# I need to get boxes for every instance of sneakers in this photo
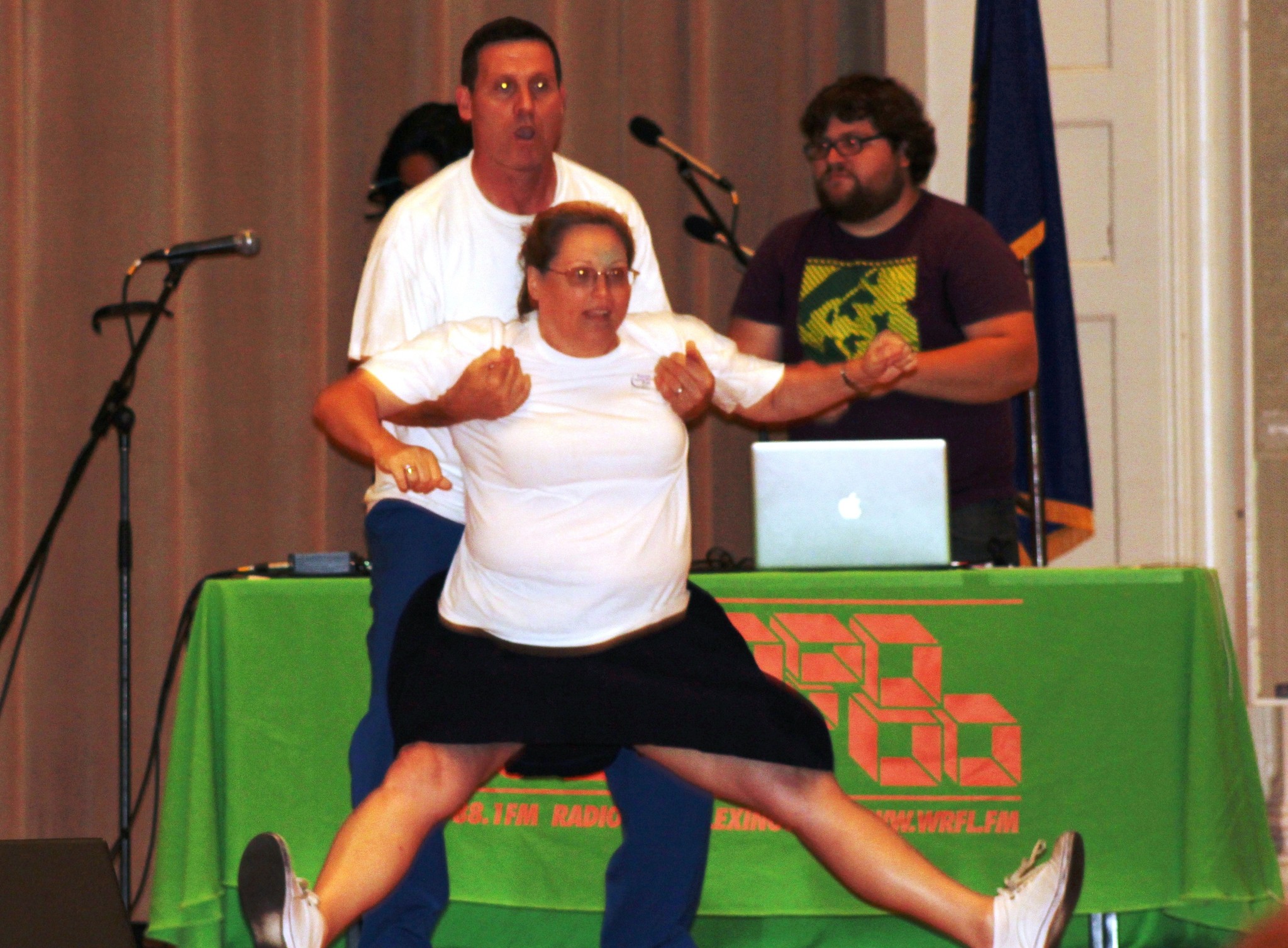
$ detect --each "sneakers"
[989,830,1083,947]
[240,831,329,948]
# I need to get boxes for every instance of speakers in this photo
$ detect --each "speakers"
[0,837,143,948]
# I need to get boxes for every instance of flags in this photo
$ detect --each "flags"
[965,0,1094,565]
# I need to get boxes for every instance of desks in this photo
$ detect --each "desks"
[143,569,1285,948]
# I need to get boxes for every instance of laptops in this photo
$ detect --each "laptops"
[749,437,965,569]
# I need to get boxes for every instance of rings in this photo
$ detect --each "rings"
[675,386,685,395]
[406,468,417,473]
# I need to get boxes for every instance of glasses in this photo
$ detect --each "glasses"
[469,77,554,99]
[801,132,882,161]
[541,266,639,290]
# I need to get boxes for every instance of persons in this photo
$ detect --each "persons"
[348,20,714,948]
[726,74,1038,564]
[239,201,1086,948]
[378,102,475,200]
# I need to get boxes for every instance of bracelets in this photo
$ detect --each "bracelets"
[843,368,863,396]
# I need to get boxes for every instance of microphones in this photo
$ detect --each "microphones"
[137,231,262,265]
[679,215,753,260]
[626,113,736,194]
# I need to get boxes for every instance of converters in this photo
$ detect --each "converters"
[291,549,364,576]
